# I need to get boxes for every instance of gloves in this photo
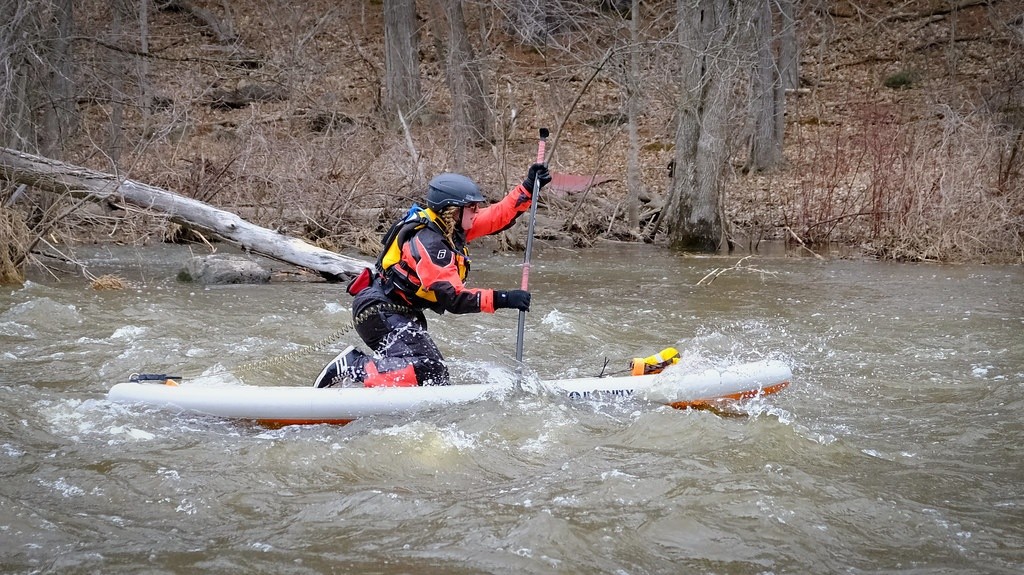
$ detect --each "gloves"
[494,289,531,312]
[523,162,553,192]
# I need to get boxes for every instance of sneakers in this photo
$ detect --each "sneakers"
[314,345,364,388]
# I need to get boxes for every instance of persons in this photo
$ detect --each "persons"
[302,156,553,387]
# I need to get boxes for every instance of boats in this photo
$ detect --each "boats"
[107,347,792,432]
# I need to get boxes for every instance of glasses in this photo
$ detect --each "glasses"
[466,201,480,212]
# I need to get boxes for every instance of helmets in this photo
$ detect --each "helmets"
[428,171,486,211]
